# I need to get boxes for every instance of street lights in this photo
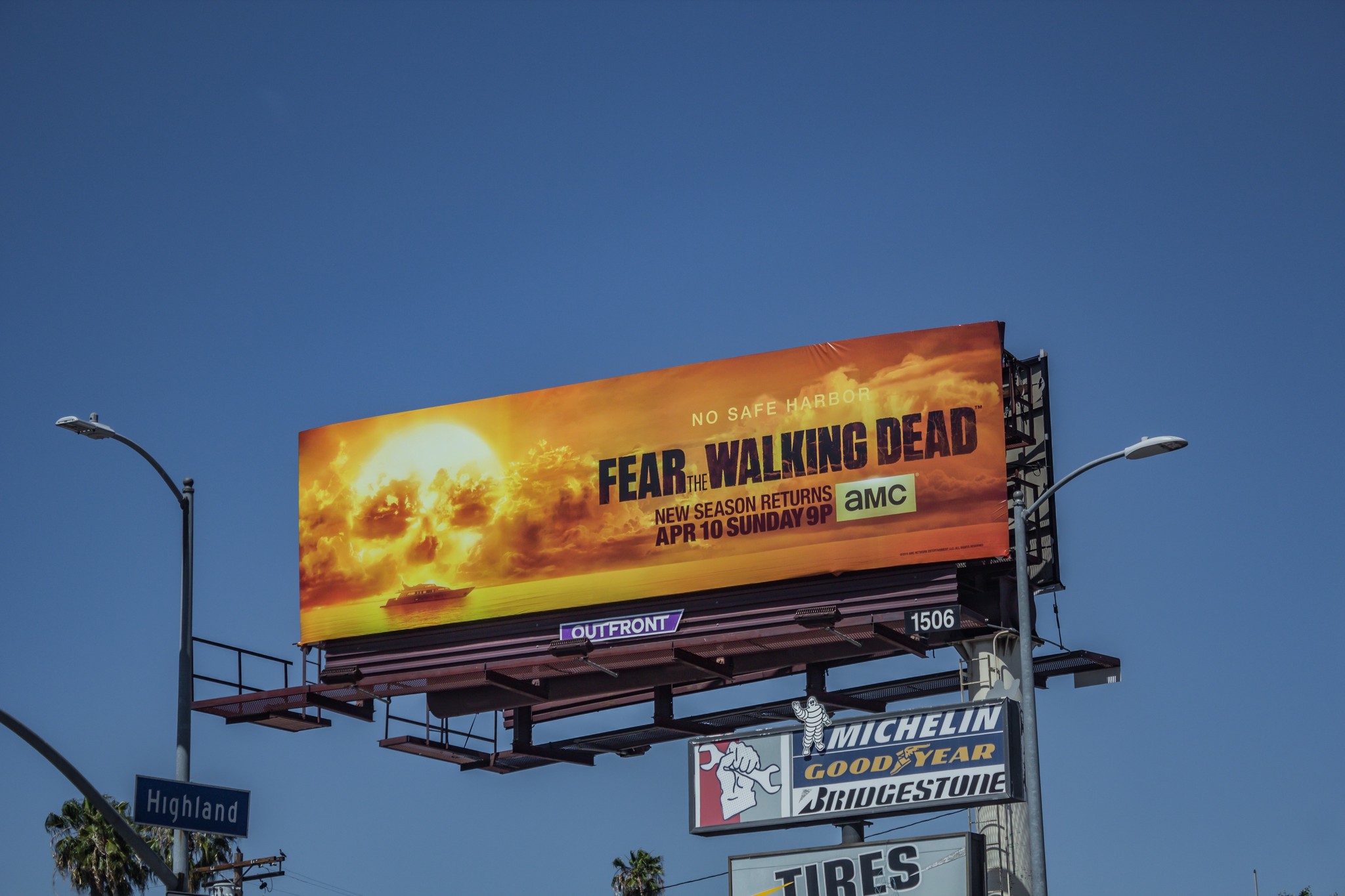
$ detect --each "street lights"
[55,413,199,896]
[1015,433,1193,896]
